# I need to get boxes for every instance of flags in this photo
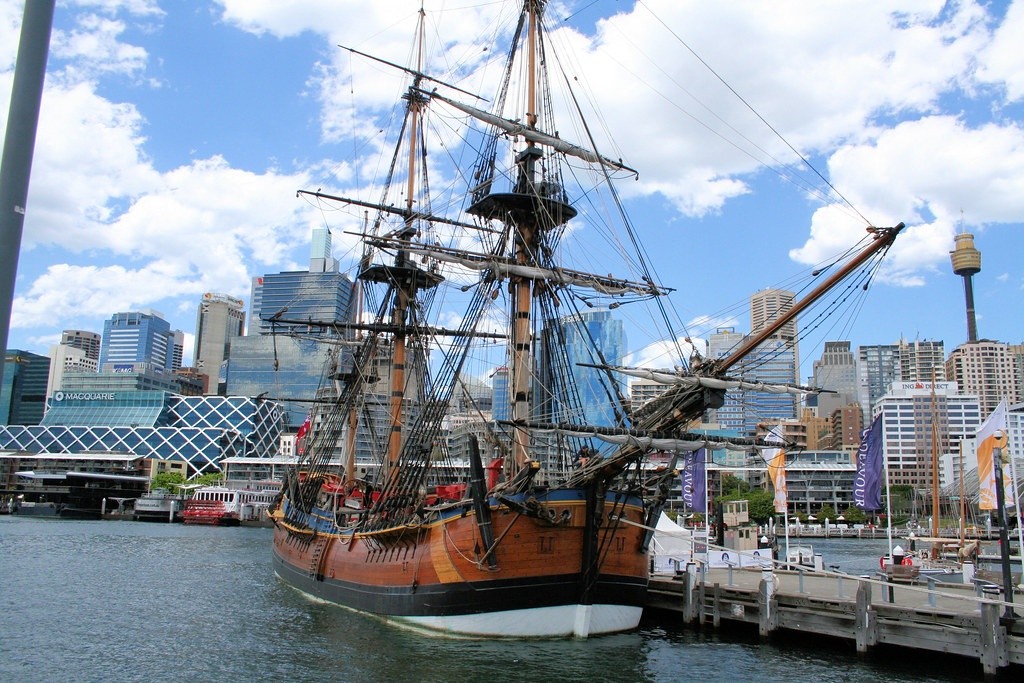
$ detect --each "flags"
[975,399,1015,510]
[682,448,705,512]
[762,424,787,506]
[853,412,883,511]
[295,407,311,446]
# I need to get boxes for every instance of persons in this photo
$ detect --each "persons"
[573,445,589,469]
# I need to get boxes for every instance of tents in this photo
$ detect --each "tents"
[648,511,691,578]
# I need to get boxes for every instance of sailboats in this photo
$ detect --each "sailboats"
[264,0,907,640]
[879,364,1021,595]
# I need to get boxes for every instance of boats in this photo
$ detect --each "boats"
[133,486,183,523]
[182,485,281,525]
[778,541,828,573]
[715,500,779,563]
